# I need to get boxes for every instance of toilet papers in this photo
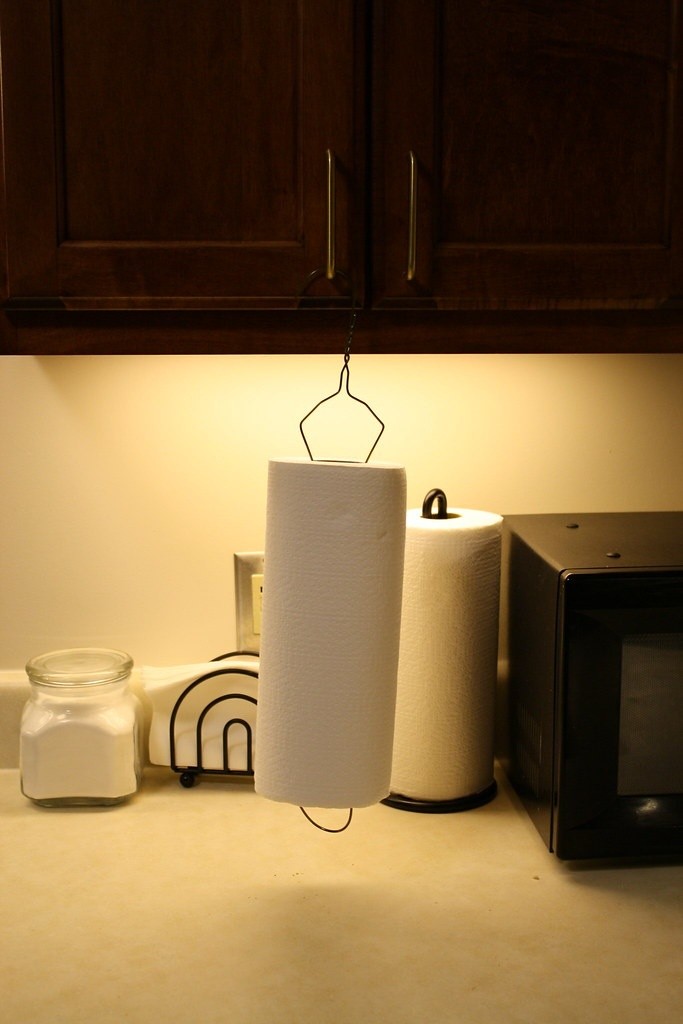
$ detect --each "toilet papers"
[389,507,504,800]
[255,458,406,809]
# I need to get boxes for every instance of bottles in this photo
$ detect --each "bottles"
[17,649,141,807]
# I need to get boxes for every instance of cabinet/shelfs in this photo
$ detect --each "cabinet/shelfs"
[0,0,682,354]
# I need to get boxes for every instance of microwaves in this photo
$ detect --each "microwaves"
[502,515,683,861]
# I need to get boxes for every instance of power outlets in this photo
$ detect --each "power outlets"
[233,552,265,651]
